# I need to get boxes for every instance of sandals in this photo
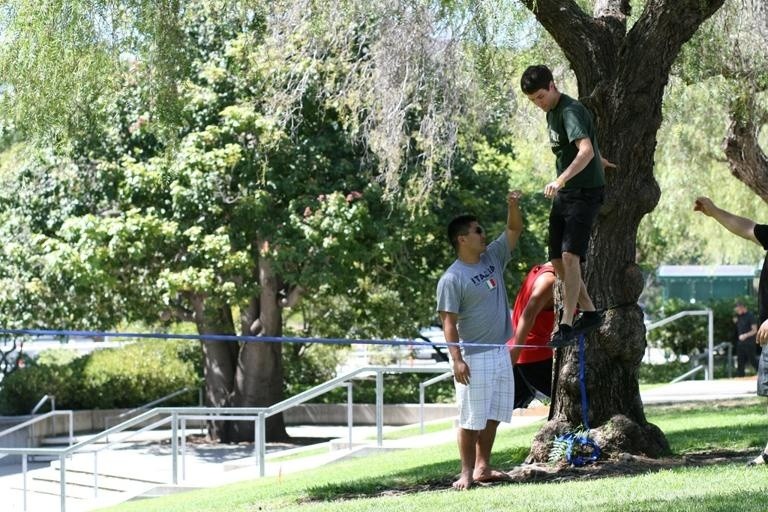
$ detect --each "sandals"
[745,451,768,467]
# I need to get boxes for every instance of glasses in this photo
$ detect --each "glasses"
[455,226,482,239]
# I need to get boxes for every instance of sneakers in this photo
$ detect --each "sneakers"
[545,324,578,347]
[567,310,602,336]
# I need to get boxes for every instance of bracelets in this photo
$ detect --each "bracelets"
[555,179,566,188]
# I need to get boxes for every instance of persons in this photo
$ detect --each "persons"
[507,259,560,415]
[518,62,620,351]
[692,193,767,467]
[431,188,526,491]
[728,298,761,379]
